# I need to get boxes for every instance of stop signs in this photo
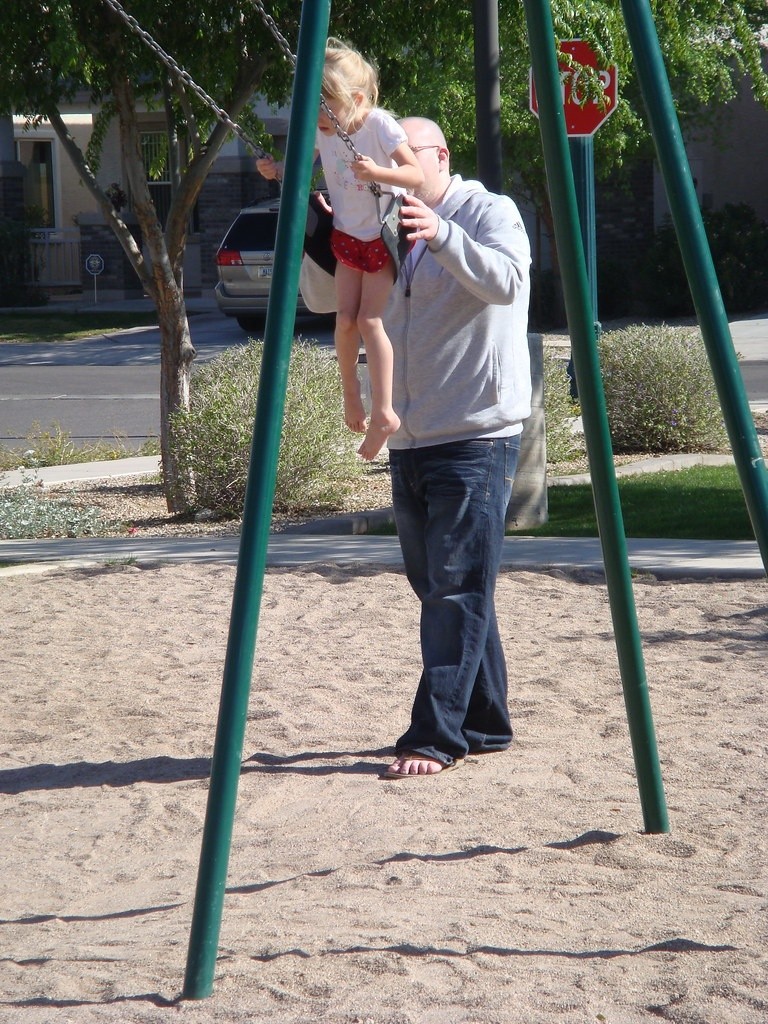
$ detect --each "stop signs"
[530,38,616,137]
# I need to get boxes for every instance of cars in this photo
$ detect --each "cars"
[214,191,337,334]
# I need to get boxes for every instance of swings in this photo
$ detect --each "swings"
[102,0,419,287]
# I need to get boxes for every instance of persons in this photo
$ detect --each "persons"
[253,45,424,461]
[381,113,536,776]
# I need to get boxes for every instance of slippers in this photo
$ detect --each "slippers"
[383,755,466,779]
